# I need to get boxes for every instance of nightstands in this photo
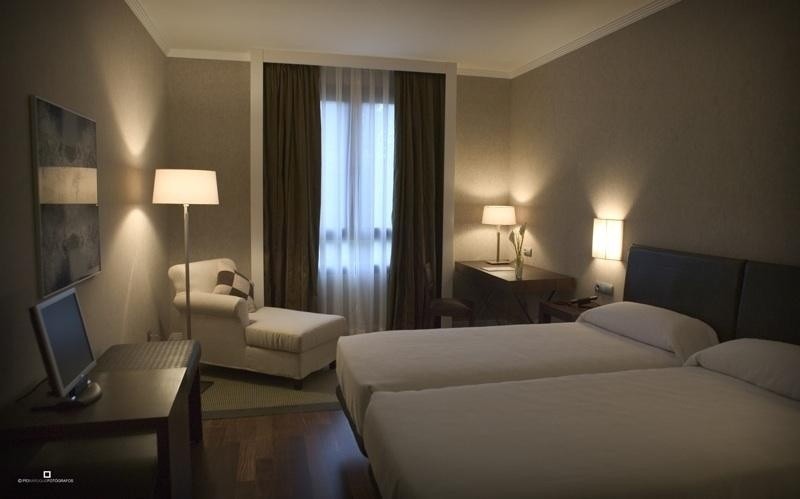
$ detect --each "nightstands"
[540,294,599,323]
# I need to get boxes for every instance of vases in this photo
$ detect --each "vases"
[513,254,524,280]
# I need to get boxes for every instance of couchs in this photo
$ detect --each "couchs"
[165,257,345,390]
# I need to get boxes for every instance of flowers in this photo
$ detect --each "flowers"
[509,221,533,270]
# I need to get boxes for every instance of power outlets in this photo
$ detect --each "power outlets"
[594,280,602,294]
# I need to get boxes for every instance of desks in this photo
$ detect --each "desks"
[452,259,577,326]
[3,339,204,499]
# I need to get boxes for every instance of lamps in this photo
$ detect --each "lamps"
[38,166,99,208]
[151,168,220,340]
[591,216,624,261]
[481,205,517,266]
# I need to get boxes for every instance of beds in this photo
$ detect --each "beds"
[362,258,800,497]
[327,247,800,456]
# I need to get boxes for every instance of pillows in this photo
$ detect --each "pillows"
[209,258,257,314]
[574,301,720,360]
[683,336,800,401]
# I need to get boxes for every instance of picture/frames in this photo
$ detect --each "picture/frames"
[30,95,103,299]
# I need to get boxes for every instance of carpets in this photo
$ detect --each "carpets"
[196,362,344,419]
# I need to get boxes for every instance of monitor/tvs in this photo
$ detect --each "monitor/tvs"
[29,286,102,410]
